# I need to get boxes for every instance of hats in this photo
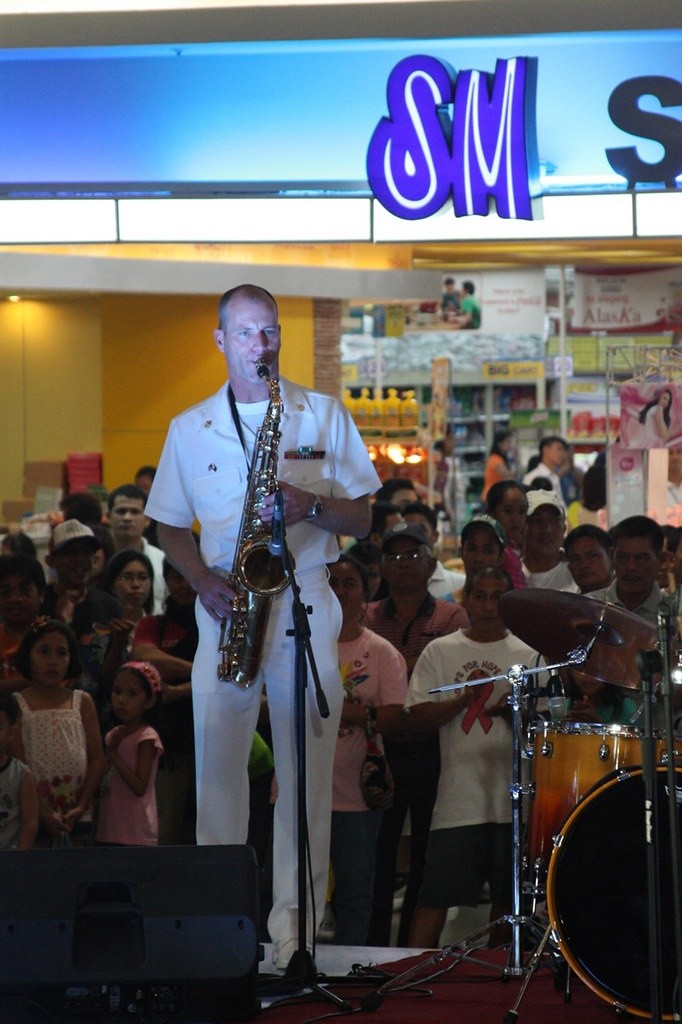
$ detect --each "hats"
[52,519,101,555]
[461,513,507,546]
[378,477,414,497]
[381,522,427,554]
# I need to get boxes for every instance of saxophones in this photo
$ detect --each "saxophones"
[215,361,295,690]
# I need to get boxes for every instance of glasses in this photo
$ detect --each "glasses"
[385,551,426,560]
[616,551,655,565]
[119,573,151,582]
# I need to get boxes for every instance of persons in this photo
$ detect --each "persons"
[143,284,383,972]
[0,429,682,950]
[627,389,673,449]
[441,277,480,330]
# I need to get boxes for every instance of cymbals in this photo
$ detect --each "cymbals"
[497,587,682,691]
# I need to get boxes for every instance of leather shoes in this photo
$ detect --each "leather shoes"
[271,937,297,969]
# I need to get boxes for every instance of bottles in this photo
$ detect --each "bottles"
[343,388,418,427]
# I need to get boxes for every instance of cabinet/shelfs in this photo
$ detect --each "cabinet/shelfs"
[354,413,514,563]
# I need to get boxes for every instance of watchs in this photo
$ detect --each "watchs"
[303,494,323,522]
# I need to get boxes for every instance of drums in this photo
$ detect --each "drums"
[520,721,682,896]
[545,762,682,1021]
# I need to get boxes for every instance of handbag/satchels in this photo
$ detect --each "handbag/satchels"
[360,703,394,811]
